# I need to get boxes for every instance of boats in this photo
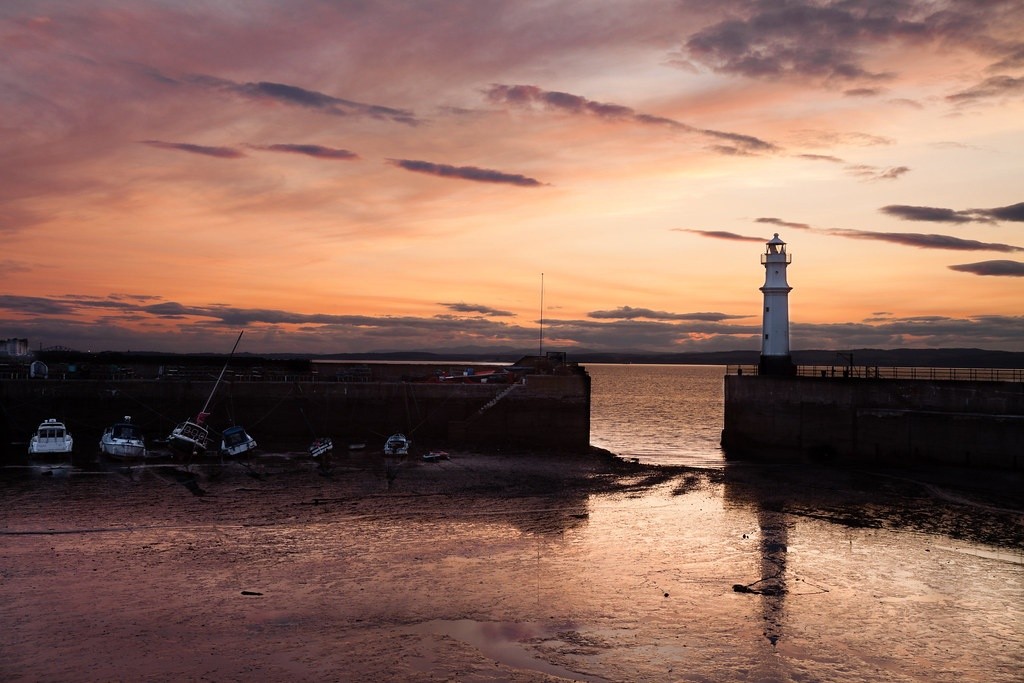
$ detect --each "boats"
[30,360,48,378]
[27,418,74,464]
[422,451,450,461]
[439,373,508,382]
[99,415,146,462]
[384,433,409,457]
[221,425,258,459]
[310,437,333,457]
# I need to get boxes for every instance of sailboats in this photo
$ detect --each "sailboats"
[164,330,244,462]
